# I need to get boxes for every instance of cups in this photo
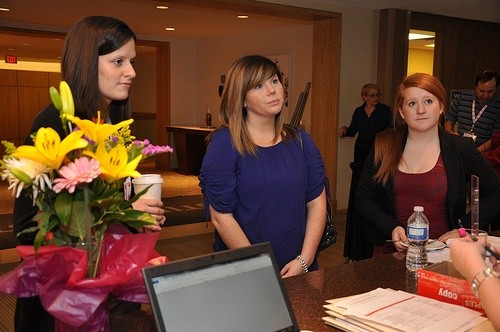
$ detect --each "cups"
[459,229,488,261]
[132,173,163,217]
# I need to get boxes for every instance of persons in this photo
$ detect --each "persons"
[197,54,331,279]
[356,70,500,260]
[12,15,166,332]
[338,83,398,163]
[450,235,500,332]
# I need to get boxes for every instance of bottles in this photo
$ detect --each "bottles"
[206,108,212,127]
[404,206,429,271]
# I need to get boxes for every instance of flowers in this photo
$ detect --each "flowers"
[0,81,172,253]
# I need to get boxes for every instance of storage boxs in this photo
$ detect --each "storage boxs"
[417,260,485,314]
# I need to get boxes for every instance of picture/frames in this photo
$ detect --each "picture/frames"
[266,51,292,108]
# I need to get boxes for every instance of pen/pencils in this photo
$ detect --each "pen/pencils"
[386,239,401,244]
[468,234,500,259]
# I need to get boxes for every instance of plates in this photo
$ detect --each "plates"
[399,238,447,251]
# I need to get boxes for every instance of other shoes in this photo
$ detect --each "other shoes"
[340,257,358,264]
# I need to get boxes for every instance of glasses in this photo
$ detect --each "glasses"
[366,93,381,97]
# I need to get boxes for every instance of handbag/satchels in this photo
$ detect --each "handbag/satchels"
[282,123,337,251]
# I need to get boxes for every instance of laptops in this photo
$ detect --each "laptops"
[140,241,301,332]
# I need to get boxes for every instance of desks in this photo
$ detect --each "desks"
[167,125,216,175]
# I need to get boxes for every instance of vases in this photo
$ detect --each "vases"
[66,242,102,278]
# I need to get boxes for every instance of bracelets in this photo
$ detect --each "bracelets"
[296,255,309,274]
[471,267,492,299]
